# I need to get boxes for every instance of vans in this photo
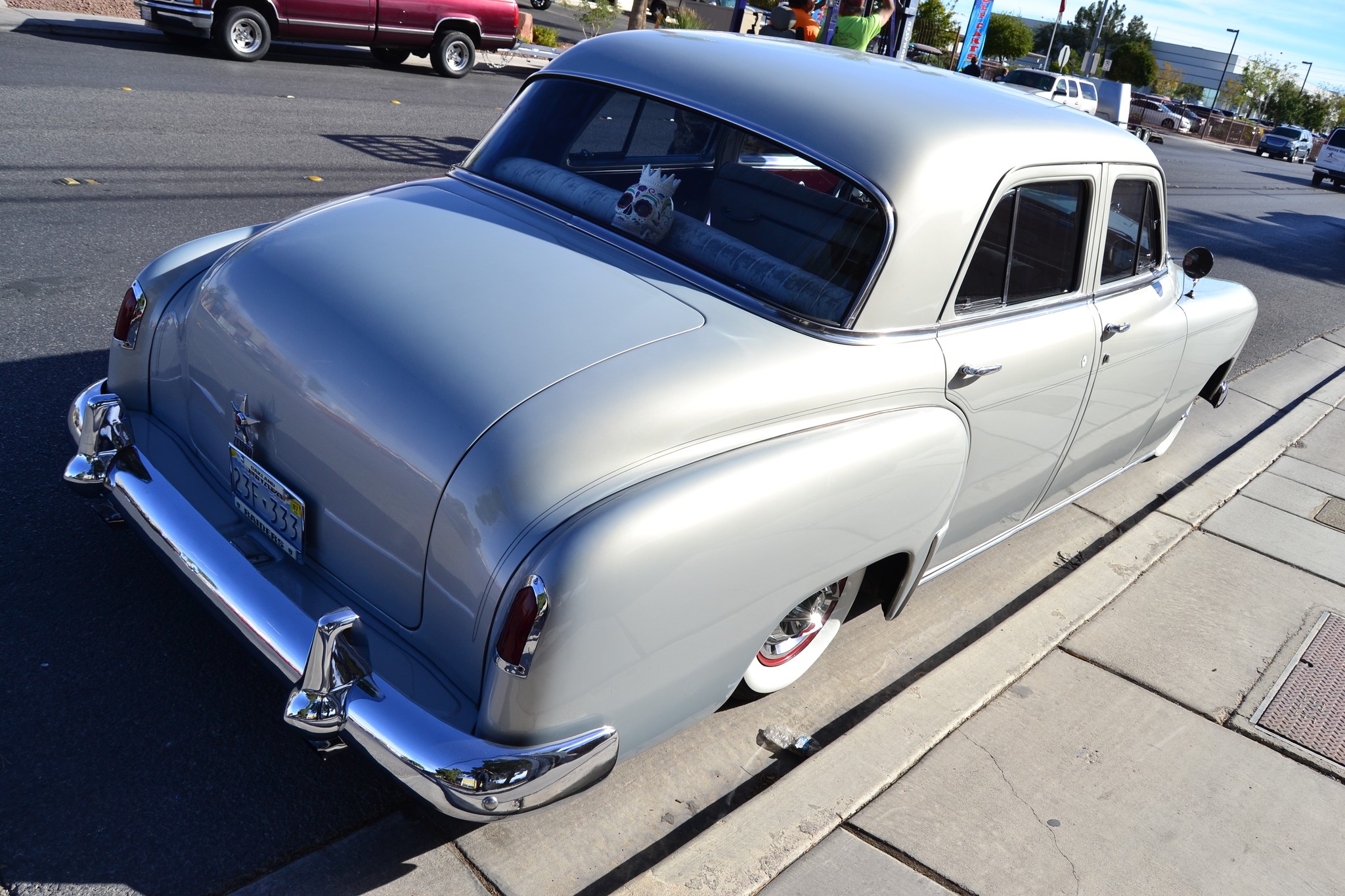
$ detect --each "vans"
[1311,127,1345,188]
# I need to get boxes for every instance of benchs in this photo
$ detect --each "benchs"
[491,155,856,328]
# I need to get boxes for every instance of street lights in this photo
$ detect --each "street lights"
[1201,29,1239,138]
[1297,61,1312,97]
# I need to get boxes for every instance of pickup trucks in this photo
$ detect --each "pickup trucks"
[647,0,771,33]
[134,0,534,79]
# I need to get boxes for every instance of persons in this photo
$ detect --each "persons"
[769,0,895,52]
[963,57,981,78]
[992,68,1009,82]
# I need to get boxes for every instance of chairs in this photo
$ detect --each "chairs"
[708,160,884,297]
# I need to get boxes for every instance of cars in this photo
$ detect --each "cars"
[64,30,1259,824]
[1129,92,1324,139]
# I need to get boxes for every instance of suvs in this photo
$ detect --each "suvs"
[1255,126,1313,164]
[996,68,1098,116]
